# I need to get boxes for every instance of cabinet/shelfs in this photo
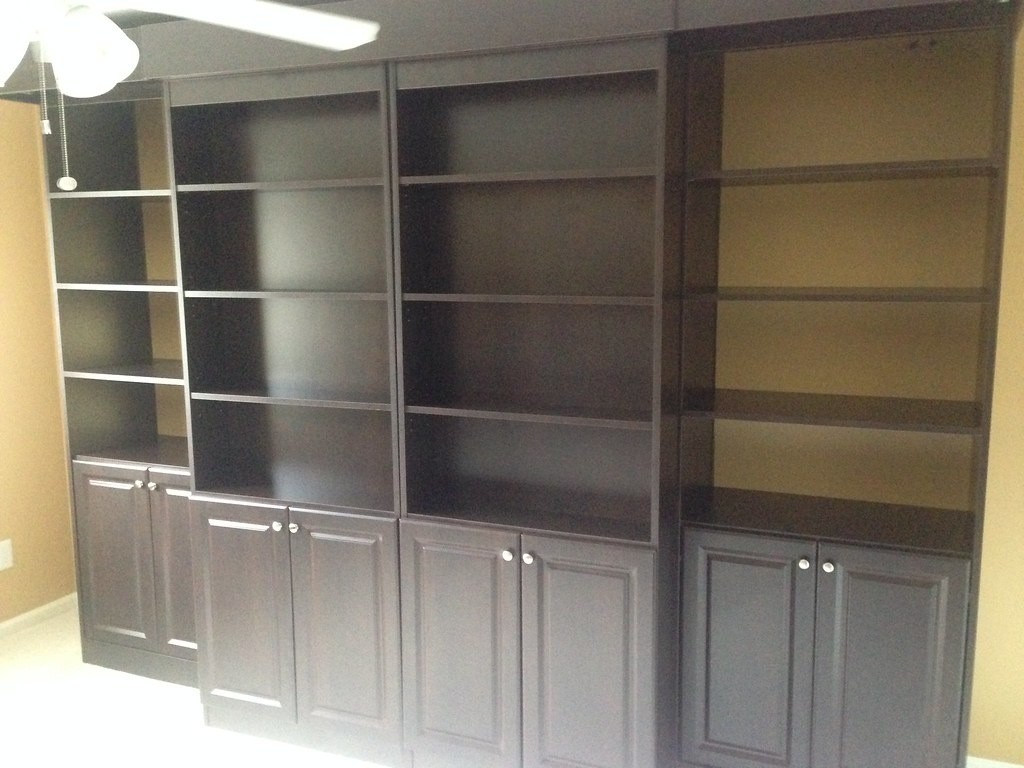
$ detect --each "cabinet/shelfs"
[163,57,397,758]
[38,75,199,688]
[676,4,1016,766]
[388,26,678,766]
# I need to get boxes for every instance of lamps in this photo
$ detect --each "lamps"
[1,2,382,100]
